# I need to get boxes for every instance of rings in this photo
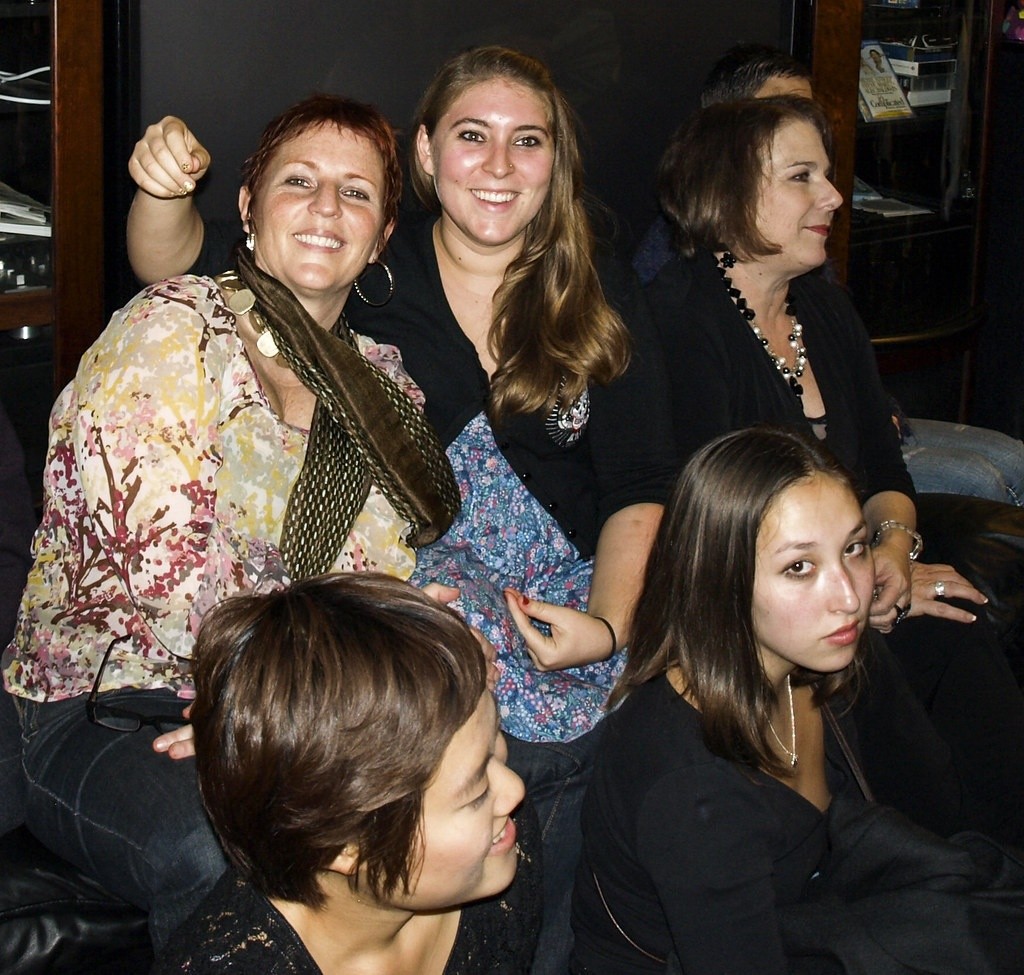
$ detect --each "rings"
[893,603,911,628]
[935,581,945,597]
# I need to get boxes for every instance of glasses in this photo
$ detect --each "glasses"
[85,633,193,735]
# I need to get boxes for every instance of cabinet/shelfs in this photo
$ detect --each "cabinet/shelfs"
[792,0,1000,424]
[0,0,103,419]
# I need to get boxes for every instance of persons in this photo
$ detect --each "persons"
[126,44,684,975]
[162,570,569,975]
[0,91,424,975]
[632,55,989,630]
[532,425,875,975]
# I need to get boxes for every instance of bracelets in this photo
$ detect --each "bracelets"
[873,585,880,600]
[595,616,616,663]
[869,519,924,563]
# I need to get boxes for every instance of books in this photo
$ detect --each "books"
[0,182,52,237]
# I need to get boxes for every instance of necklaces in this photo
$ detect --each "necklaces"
[711,256,809,396]
[761,674,799,769]
[219,269,290,369]
